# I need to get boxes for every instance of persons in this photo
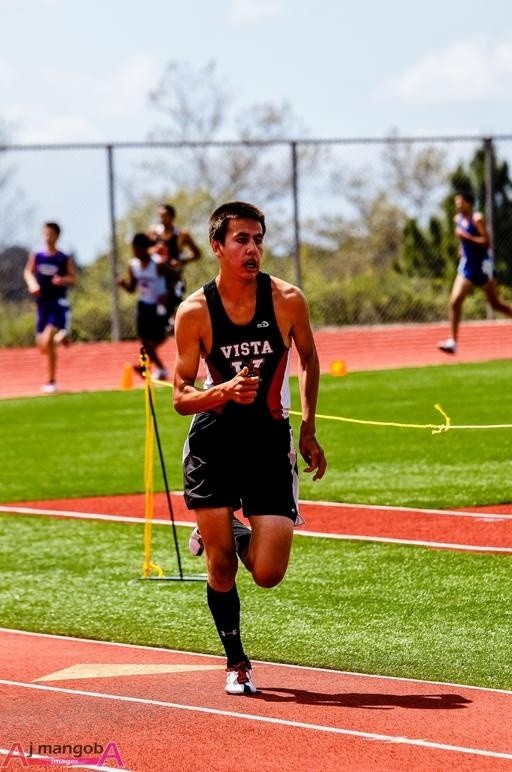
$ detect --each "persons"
[437,188,512,353]
[174,201,327,695]
[22,222,78,395]
[147,204,202,334]
[117,232,185,380]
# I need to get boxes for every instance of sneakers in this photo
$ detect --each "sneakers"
[226,661,256,694]
[189,527,204,556]
[439,338,456,354]
